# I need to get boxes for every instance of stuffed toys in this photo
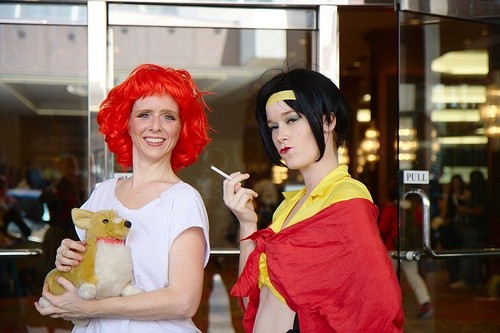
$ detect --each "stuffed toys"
[38,208,146,327]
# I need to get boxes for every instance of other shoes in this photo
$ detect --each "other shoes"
[417,301,435,323]
[450,278,470,290]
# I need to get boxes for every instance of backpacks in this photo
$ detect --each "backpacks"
[392,198,425,253]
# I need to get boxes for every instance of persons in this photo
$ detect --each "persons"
[0,157,87,237]
[223,68,402,333]
[444,171,488,239]
[378,181,435,318]
[35,64,218,333]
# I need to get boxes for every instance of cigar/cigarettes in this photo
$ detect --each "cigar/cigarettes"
[209,165,242,186]
